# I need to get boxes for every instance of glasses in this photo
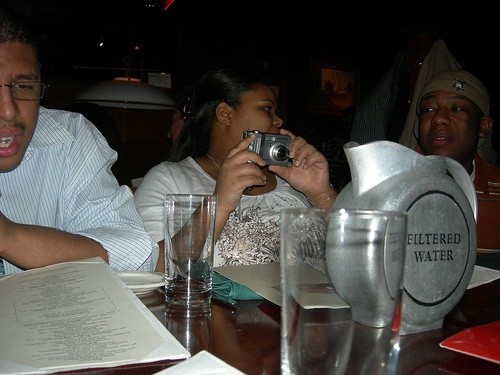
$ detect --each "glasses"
[1,80,49,99]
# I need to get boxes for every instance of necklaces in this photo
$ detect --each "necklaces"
[206,153,222,169]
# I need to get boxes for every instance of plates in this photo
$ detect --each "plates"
[118,271,165,295]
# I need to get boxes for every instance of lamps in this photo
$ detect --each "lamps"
[72,76,177,111]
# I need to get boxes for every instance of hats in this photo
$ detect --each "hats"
[416,69,491,118]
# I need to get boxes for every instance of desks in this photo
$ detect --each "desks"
[1,241,500,375]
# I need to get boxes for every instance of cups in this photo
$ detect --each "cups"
[164,192,216,319]
[279,208,407,375]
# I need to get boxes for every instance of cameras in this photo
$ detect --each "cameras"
[241,130,294,167]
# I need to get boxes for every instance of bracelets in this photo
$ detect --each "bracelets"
[306,184,333,206]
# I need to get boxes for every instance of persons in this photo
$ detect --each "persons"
[135,57,337,273]
[414,69,500,249]
[0,10,159,272]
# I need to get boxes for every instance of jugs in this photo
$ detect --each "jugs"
[325,140,477,335]
